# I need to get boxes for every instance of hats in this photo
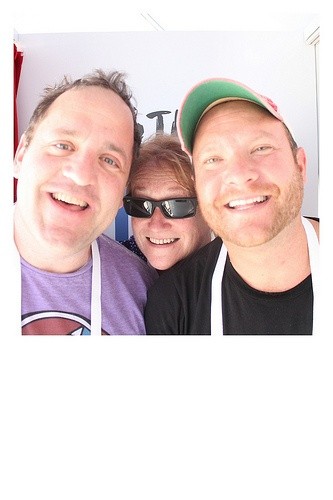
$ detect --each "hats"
[176,76,283,163]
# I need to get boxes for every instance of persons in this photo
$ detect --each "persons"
[142,78,320,337]
[14,68,158,335]
[120,129,215,272]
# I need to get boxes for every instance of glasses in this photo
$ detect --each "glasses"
[122,192,197,219]
